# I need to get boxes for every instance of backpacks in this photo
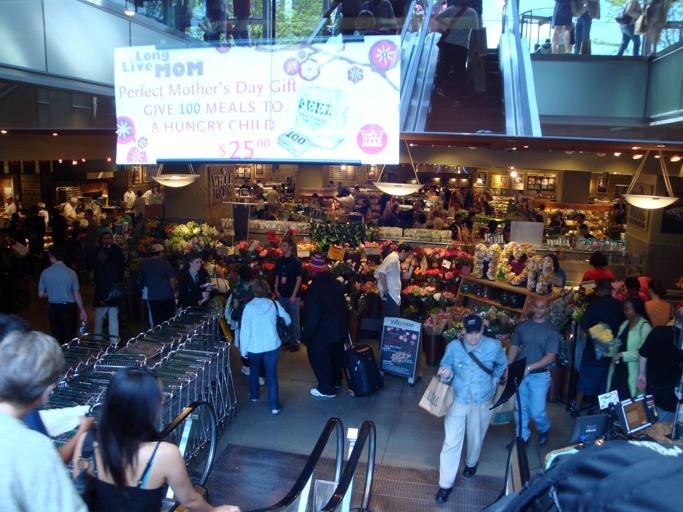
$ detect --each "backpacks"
[501,436,683,512]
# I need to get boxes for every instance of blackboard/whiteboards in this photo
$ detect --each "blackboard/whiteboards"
[380,317,422,384]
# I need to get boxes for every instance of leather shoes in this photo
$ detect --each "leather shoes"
[462,461,478,478]
[527,367,549,373]
[536,429,550,446]
[436,485,453,503]
[505,438,531,450]
[566,405,582,418]
[588,408,598,416]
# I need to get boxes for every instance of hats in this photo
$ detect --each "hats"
[151,243,165,253]
[307,253,326,273]
[464,314,481,334]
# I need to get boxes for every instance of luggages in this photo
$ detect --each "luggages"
[344,330,384,400]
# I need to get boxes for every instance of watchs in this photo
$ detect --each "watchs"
[528,365,532,372]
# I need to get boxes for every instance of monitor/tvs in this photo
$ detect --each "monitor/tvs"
[616,394,659,440]
[597,390,619,410]
[569,415,608,451]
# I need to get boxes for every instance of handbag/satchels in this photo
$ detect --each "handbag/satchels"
[418,372,452,418]
[272,298,300,354]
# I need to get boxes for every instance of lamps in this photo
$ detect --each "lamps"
[621,148,679,212]
[373,139,426,198]
[153,163,201,188]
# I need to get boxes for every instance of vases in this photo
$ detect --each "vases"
[423,300,432,310]
[406,297,416,306]
[370,296,380,316]
[348,314,360,343]
[426,335,444,368]
[124,296,138,321]
[548,368,562,403]
[139,297,147,322]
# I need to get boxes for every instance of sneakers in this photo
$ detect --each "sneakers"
[270,407,280,417]
[248,392,256,402]
[310,388,336,398]
[241,365,251,377]
[258,376,266,386]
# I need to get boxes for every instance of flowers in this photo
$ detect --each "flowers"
[337,276,367,314]
[164,219,234,280]
[588,321,622,347]
[124,257,144,296]
[526,255,554,294]
[499,241,536,285]
[418,286,435,297]
[546,297,573,369]
[234,238,315,275]
[448,305,517,335]
[422,307,451,336]
[361,281,379,294]
[401,286,419,296]
[472,243,502,280]
[346,237,397,273]
[103,271,139,302]
[434,291,458,306]
[403,248,472,285]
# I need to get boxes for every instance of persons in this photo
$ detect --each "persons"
[354,0,400,36]
[48,207,69,246]
[569,278,623,415]
[0,330,89,512]
[91,250,120,350]
[625,277,653,327]
[545,254,566,286]
[642,0,669,56]
[72,368,243,512]
[62,197,78,225]
[224,267,265,385]
[116,208,132,225]
[239,278,292,415]
[123,187,137,202]
[201,249,226,295]
[606,297,652,399]
[436,3,478,107]
[0,313,94,464]
[0,204,4,216]
[574,0,600,54]
[507,192,532,221]
[72,219,88,239]
[149,187,158,199]
[86,219,114,242]
[550,0,572,54]
[38,247,87,349]
[532,197,626,240]
[231,0,253,45]
[299,253,349,398]
[373,242,413,350]
[240,174,295,221]
[352,11,374,37]
[636,308,683,423]
[178,252,213,310]
[415,183,498,242]
[24,205,46,237]
[199,0,231,53]
[84,209,97,233]
[298,181,347,224]
[75,200,86,212]
[91,194,102,209]
[435,314,507,505]
[346,185,414,229]
[645,280,674,325]
[323,0,358,34]
[5,194,16,214]
[446,0,487,94]
[139,243,176,327]
[499,295,559,451]
[37,201,49,230]
[89,232,124,274]
[616,0,643,56]
[536,39,550,54]
[274,237,304,352]
[582,251,614,282]
[132,190,146,217]
[17,199,23,210]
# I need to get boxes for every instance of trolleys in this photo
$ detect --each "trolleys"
[36,310,240,466]
